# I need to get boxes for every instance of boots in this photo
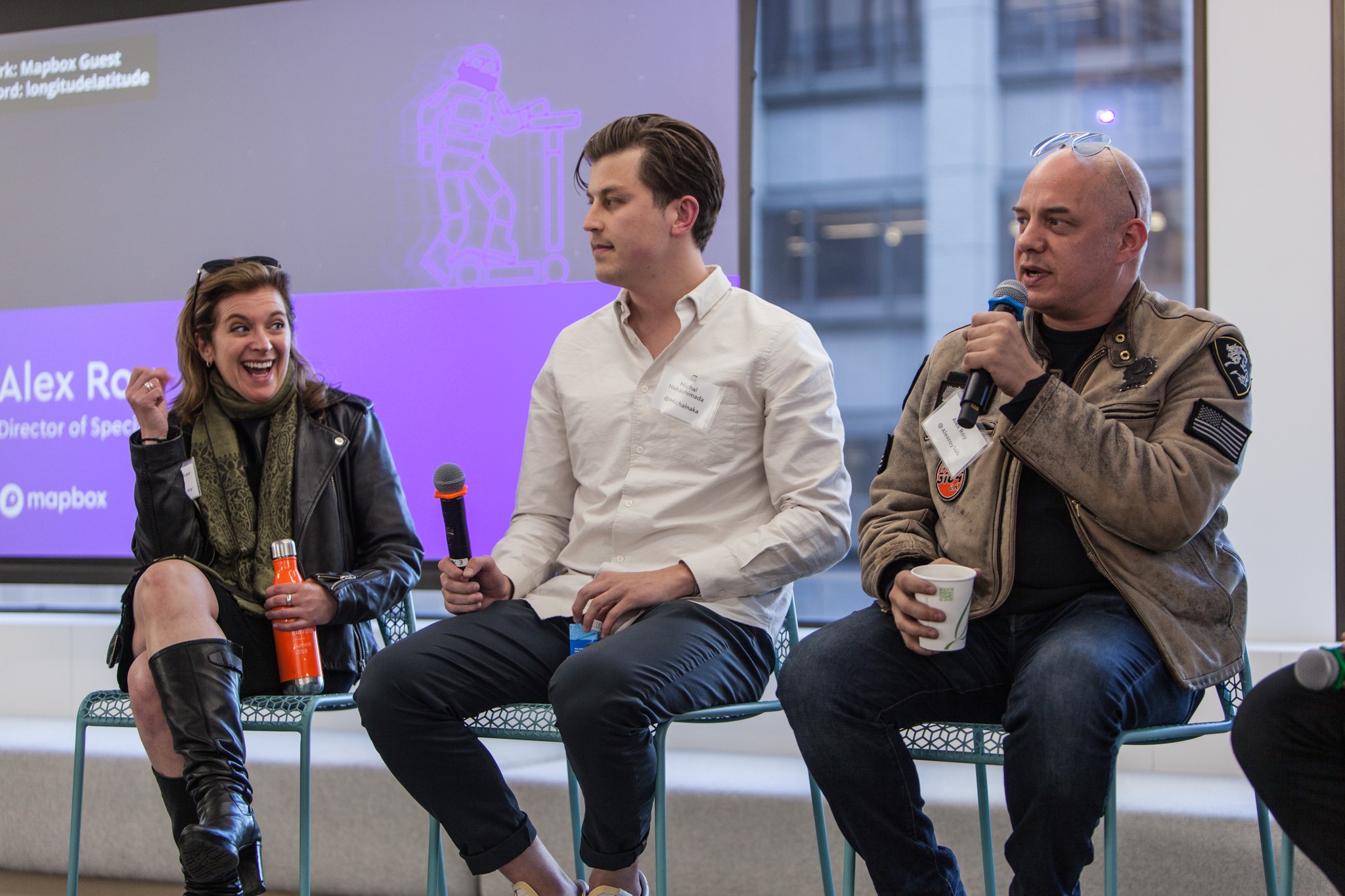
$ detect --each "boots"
[151,766,247,896]
[147,639,267,896]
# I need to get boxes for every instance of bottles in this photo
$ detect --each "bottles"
[270,538,325,696]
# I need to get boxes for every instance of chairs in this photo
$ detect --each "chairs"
[839,636,1281,896]
[67,591,447,896]
[424,589,838,896]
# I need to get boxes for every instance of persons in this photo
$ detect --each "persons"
[776,130,1253,896]
[1231,629,1345,896]
[352,114,851,896]
[107,255,424,896]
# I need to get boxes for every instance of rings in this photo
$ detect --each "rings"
[144,381,154,391]
[287,593,291,607]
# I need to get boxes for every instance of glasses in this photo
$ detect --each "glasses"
[191,256,283,348]
[1029,129,1138,221]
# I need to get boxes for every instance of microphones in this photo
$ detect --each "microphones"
[433,463,474,583]
[957,278,1029,429]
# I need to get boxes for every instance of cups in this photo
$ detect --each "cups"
[910,564,977,652]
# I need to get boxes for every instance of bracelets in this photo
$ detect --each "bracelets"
[140,438,167,443]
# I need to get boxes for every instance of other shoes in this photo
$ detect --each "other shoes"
[511,879,588,896]
[588,869,650,896]
